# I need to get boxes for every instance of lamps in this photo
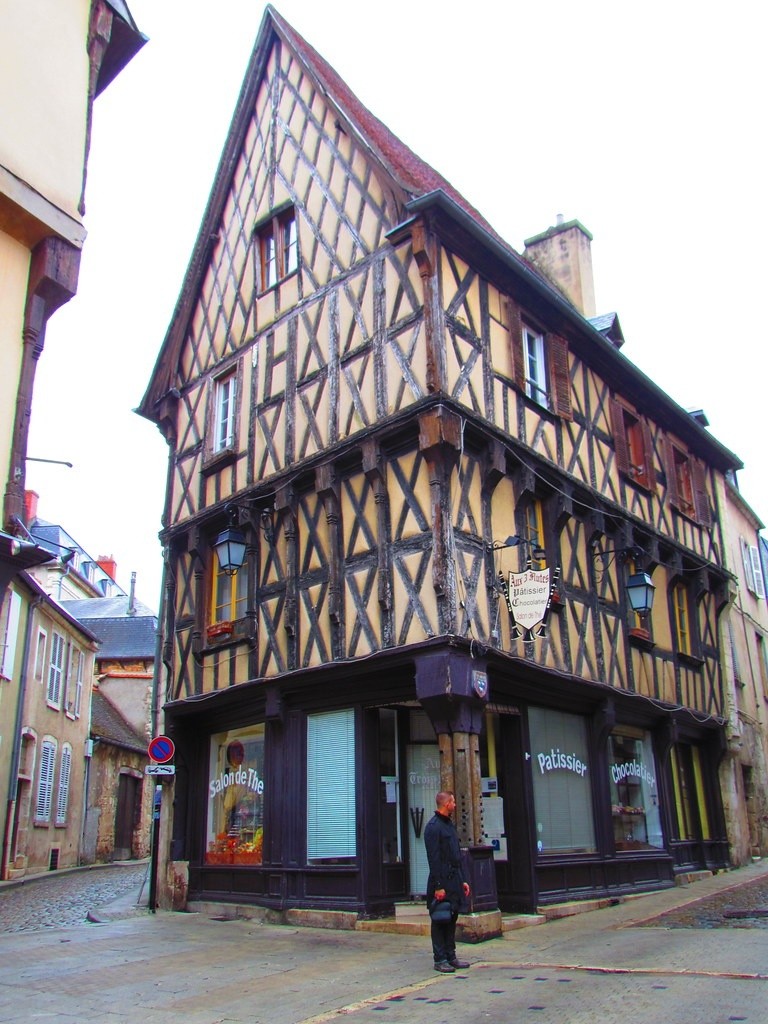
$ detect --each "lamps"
[214,503,273,577]
[592,546,657,620]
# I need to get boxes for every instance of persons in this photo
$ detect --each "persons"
[424,789,470,972]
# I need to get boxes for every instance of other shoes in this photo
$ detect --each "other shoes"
[448,959,470,968]
[434,961,454,972]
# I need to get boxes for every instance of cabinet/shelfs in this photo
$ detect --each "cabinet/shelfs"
[608,746,649,843]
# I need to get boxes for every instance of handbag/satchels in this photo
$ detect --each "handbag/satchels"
[430,897,453,924]
